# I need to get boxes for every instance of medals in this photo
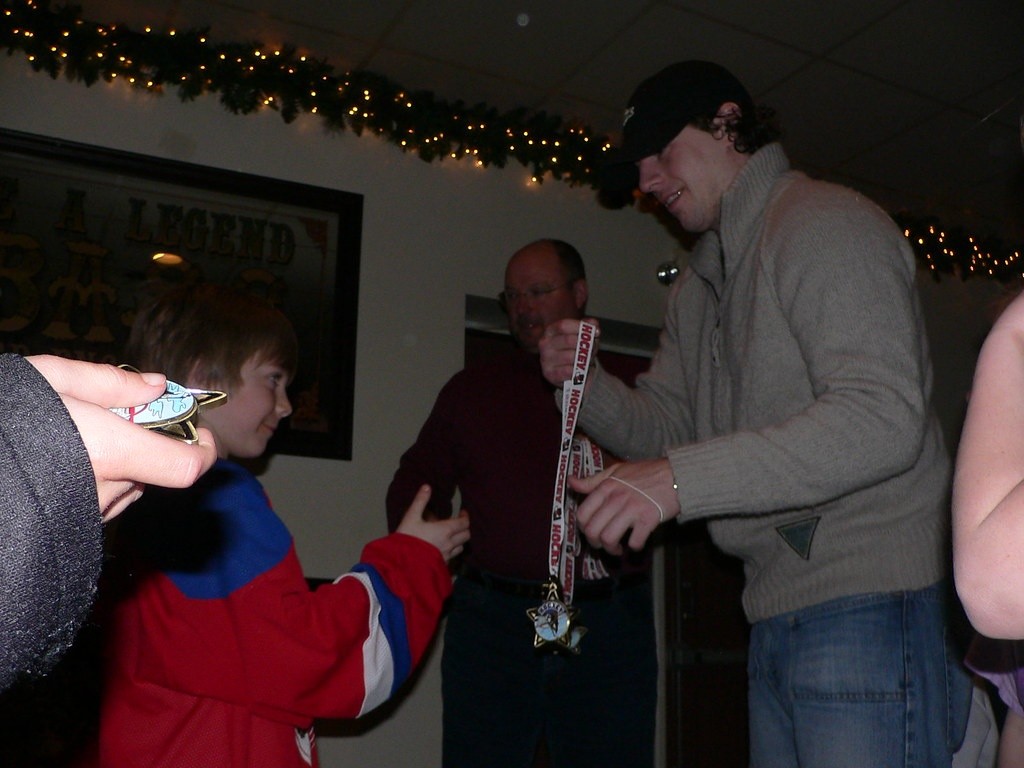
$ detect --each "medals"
[525,575,588,659]
[109,379,228,447]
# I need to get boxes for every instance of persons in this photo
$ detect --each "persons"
[536,57,956,768]
[0,350,220,708]
[385,239,658,768]
[950,275,1024,768]
[84,283,473,768]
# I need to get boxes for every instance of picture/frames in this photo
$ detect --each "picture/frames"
[0,126,364,461]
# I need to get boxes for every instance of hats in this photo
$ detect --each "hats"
[608,60,751,167]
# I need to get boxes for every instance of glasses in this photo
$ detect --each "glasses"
[496,278,579,308]
